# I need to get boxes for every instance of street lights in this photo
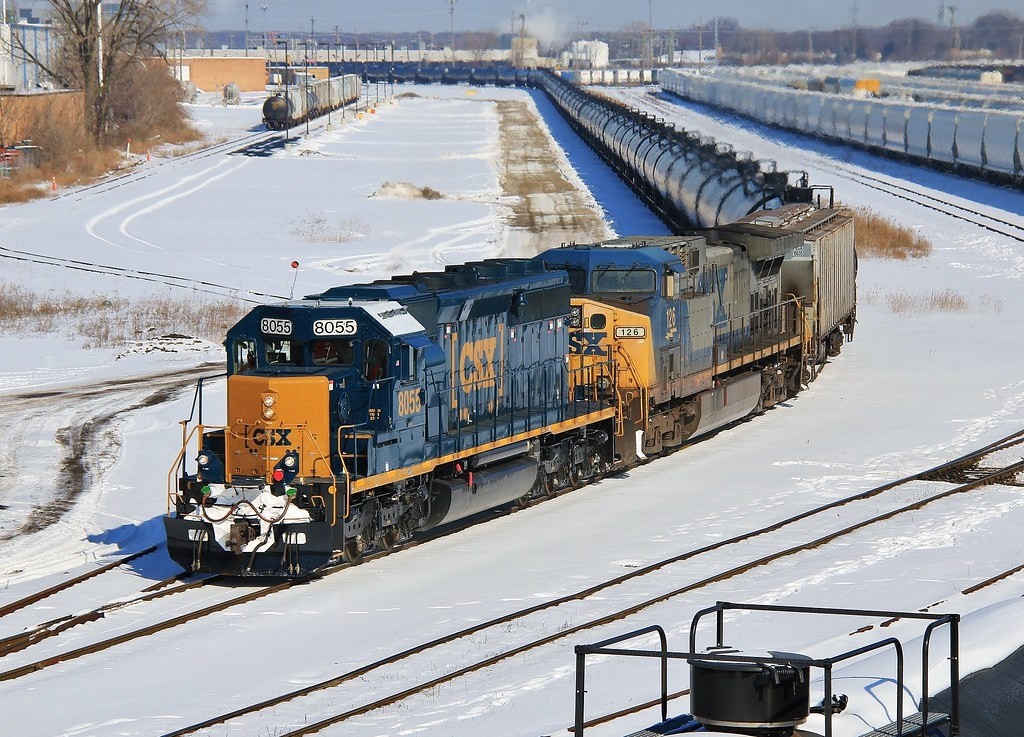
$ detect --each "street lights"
[334,42,346,125]
[260,4,268,46]
[360,42,369,112]
[319,41,332,132]
[276,41,291,152]
[349,43,359,118]
[370,43,378,102]
[297,42,311,140]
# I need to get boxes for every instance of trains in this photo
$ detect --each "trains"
[163,61,859,576]
[262,74,362,131]
